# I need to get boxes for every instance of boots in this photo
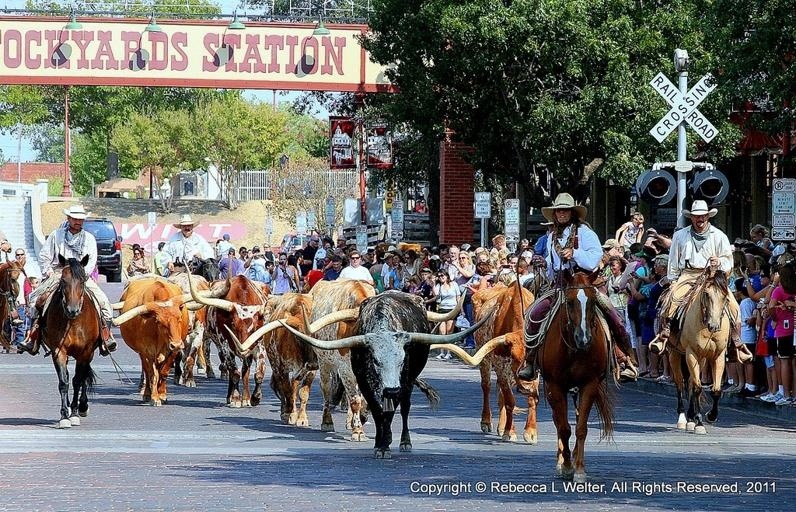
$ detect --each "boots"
[17,316,34,350]
[10,307,24,324]
[729,323,752,362]
[651,317,671,353]
[102,321,116,350]
[613,332,638,379]
[519,330,538,379]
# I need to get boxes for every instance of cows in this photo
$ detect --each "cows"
[110,252,540,459]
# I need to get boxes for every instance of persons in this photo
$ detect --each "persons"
[0,191,796,408]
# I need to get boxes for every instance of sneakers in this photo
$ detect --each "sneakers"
[639,370,796,407]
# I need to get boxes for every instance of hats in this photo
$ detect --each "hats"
[62,205,91,220]
[635,249,656,261]
[380,252,394,260]
[602,239,620,249]
[173,214,199,229]
[540,193,588,225]
[682,200,718,218]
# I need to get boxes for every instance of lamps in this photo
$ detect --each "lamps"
[228,11,245,29]
[313,14,329,36]
[145,12,160,32]
[65,8,82,29]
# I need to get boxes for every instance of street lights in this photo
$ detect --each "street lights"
[673,48,690,233]
[59,85,72,196]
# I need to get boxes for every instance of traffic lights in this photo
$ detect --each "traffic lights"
[692,169,730,206]
[639,170,677,206]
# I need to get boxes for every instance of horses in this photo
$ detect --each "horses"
[537,267,617,484]
[0,257,30,354]
[42,254,100,429]
[654,266,734,435]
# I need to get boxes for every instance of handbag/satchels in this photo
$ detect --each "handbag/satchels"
[455,315,470,328]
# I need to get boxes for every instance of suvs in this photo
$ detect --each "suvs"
[75,217,124,284]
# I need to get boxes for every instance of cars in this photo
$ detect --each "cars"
[282,179,318,199]
[281,234,314,252]
